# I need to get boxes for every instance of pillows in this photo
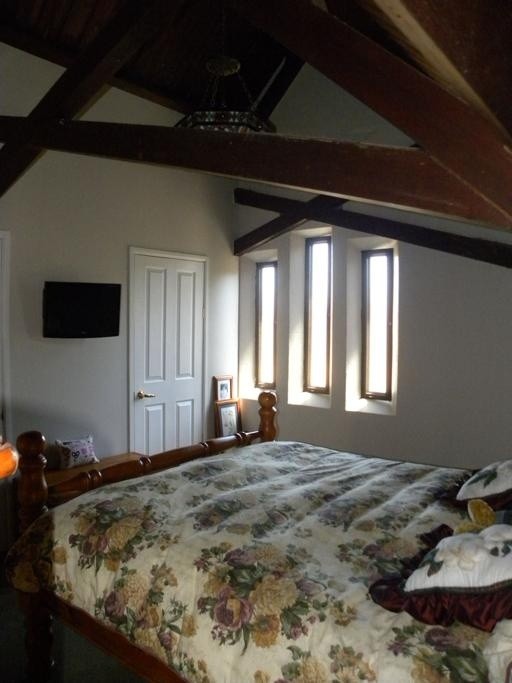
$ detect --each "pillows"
[373,518,512,628]
[56,435,97,469]
[453,458,512,510]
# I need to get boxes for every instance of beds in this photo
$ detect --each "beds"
[5,392,512,682]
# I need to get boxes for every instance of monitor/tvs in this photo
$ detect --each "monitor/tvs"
[42,281,121,338]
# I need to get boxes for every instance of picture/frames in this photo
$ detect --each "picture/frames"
[213,376,232,401]
[214,399,242,437]
